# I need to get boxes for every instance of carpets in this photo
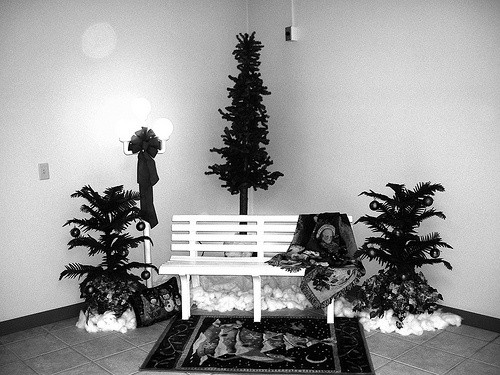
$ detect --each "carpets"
[138,309,376,375]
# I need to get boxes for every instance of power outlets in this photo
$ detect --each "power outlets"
[284,25,298,43]
[38,161,51,182]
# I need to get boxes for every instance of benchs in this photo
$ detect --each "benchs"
[157,212,355,325]
